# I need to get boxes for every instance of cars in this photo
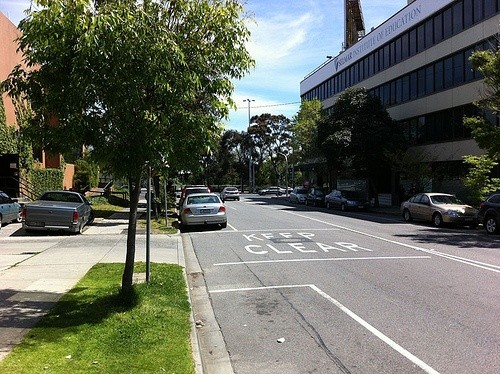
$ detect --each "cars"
[0,191,22,231]
[258,186,286,196]
[177,185,211,208]
[324,189,371,212]
[290,186,309,204]
[400,193,480,229]
[179,193,227,232]
[305,186,331,207]
[220,186,239,201]
[283,187,294,194]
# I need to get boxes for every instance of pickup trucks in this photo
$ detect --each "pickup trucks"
[21,191,95,235]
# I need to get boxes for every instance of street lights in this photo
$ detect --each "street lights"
[243,99,256,194]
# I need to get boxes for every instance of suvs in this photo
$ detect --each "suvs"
[479,193,500,235]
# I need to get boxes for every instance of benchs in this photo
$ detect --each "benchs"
[189,198,214,204]
